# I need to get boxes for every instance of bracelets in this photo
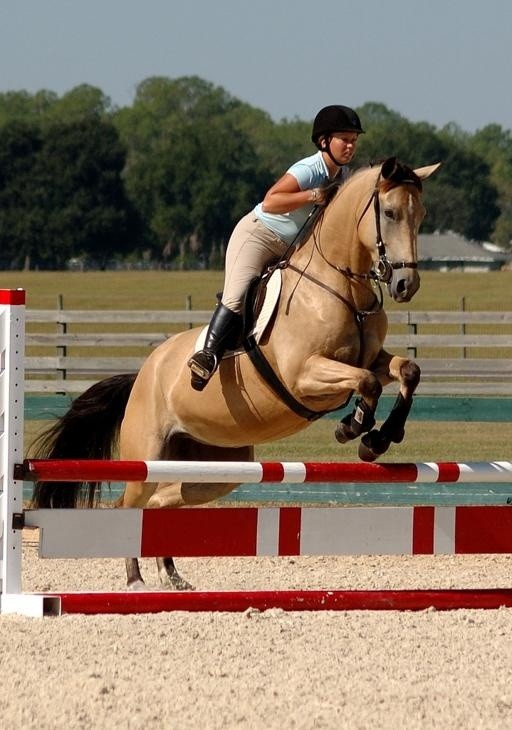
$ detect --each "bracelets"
[307,187,320,204]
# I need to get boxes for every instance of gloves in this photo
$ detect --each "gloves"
[308,176,340,208]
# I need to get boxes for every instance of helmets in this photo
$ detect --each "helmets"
[311,104,366,144]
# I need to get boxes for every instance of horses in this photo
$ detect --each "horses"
[23,156,442,591]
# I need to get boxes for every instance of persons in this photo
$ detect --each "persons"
[188,103,356,396]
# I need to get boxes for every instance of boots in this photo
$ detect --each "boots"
[190,299,242,391]
